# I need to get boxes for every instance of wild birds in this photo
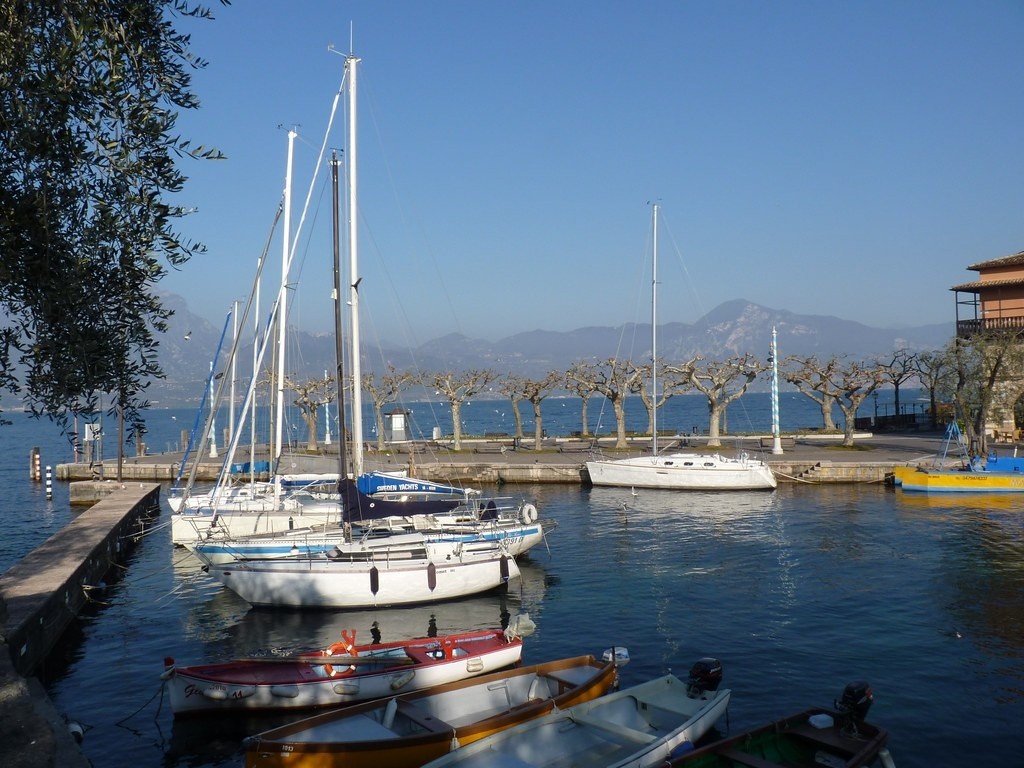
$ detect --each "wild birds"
[183,331,193,342]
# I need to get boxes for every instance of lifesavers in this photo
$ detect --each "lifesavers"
[323,641,359,677]
[522,504,538,525]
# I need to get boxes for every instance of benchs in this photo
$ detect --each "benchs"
[560,439,602,454]
[243,444,292,455]
[527,439,557,449]
[799,428,825,432]
[396,444,427,454]
[324,444,351,454]
[571,431,593,436]
[412,441,440,451]
[760,438,795,452]
[611,431,634,434]
[572,714,661,745]
[703,429,723,434]
[647,439,682,454]
[523,432,535,437]
[485,432,508,438]
[714,747,785,768]
[656,430,677,435]
[396,700,455,732]
[405,648,435,663]
[474,441,515,455]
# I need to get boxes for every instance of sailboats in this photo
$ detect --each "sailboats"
[584,196,778,490]
[167,18,559,607]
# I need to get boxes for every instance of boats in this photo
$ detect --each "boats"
[159,612,536,719]
[885,412,1023,493]
[242,645,630,768]
[653,680,896,768]
[418,656,733,768]
